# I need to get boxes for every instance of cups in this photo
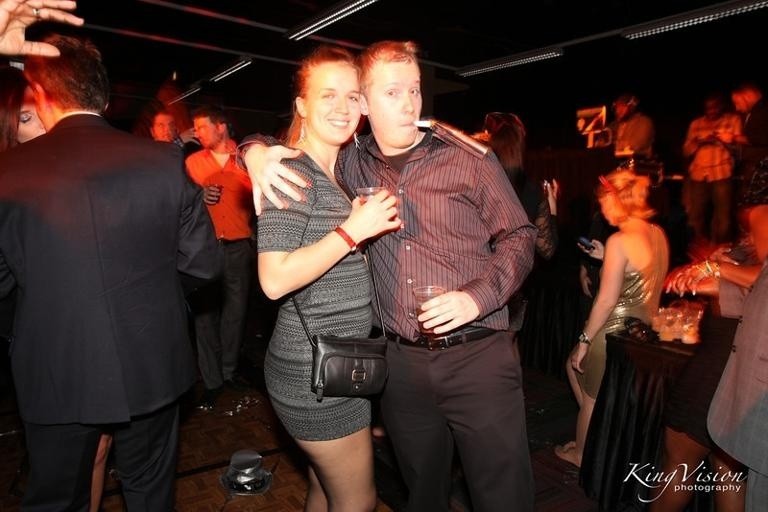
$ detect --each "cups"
[409,284,446,338]
[354,183,396,235]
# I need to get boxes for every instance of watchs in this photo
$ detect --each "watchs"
[579,332,593,347]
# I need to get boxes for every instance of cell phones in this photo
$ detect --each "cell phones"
[579,236,595,250]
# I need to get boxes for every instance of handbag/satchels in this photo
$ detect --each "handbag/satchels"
[312,335,389,397]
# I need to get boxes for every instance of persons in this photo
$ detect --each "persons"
[465,109,560,397]
[652,84,767,512]
[0,31,220,512]
[234,38,543,512]
[149,111,201,149]
[0,0,88,59]
[0,71,113,512]
[550,166,671,468]
[253,43,402,512]
[613,94,656,159]
[184,107,253,408]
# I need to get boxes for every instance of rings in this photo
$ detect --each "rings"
[34,8,43,21]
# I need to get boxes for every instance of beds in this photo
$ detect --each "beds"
[578,302,713,512]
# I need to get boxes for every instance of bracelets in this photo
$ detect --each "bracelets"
[238,143,252,169]
[335,226,359,252]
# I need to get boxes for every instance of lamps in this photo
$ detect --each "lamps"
[453,44,564,78]
[619,1,767,38]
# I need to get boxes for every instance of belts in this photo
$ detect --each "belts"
[371,325,498,348]
